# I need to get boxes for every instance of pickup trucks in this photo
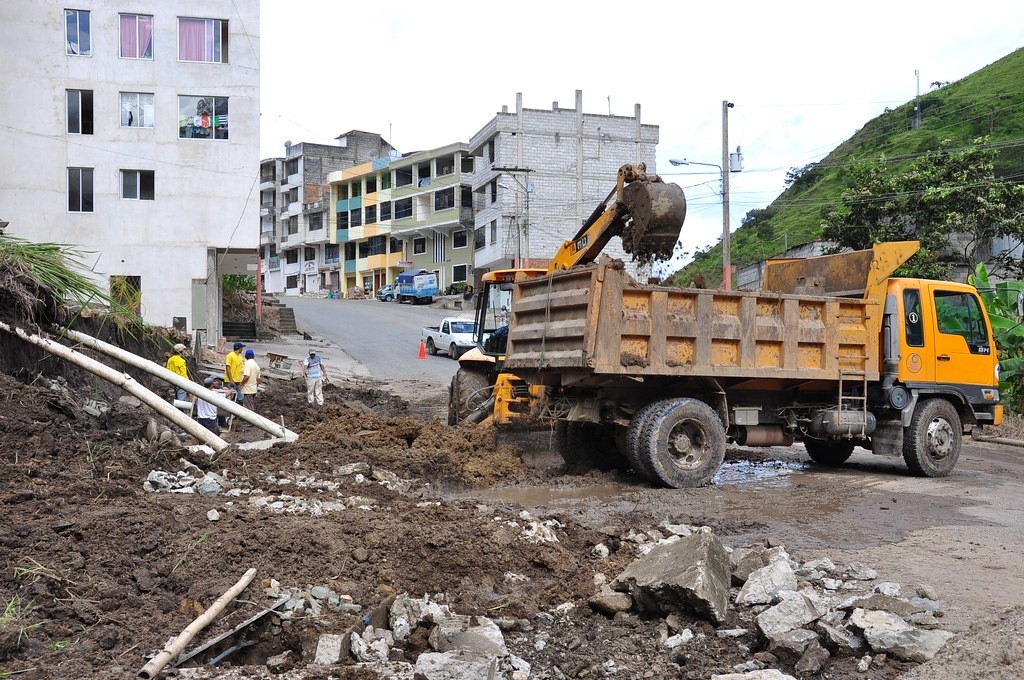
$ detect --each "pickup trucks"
[421,317,490,360]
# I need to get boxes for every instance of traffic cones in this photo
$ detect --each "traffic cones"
[416,340,428,358]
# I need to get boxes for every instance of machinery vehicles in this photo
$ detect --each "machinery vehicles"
[448,162,687,424]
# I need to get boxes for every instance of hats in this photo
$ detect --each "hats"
[309,348,316,355]
[210,375,218,380]
[174,344,186,352]
[203,378,214,386]
[233,342,246,349]
[245,349,254,357]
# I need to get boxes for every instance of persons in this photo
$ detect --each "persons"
[303,348,329,405]
[237,349,260,427]
[166,344,190,412]
[224,343,246,406]
[209,375,229,427]
[197,378,235,436]
[497,305,508,326]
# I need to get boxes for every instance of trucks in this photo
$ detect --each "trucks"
[394,269,437,305]
[503,240,1004,488]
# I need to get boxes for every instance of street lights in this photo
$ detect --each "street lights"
[498,182,529,269]
[669,158,731,293]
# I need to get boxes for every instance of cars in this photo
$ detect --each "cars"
[377,285,395,301]
